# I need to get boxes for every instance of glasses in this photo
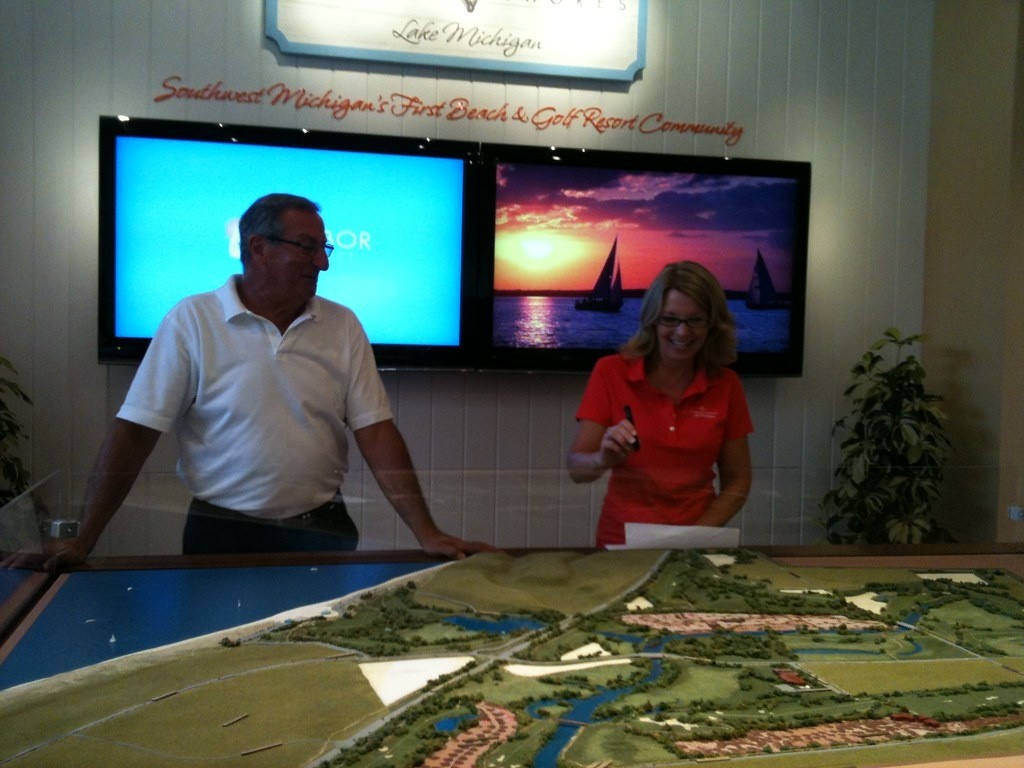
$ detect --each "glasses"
[268,237,334,259]
[656,315,712,328]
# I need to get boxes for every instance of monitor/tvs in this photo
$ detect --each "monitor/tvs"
[97,115,478,373]
[477,141,811,379]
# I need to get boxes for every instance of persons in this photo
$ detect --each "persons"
[0,193,505,571]
[567,260,752,549]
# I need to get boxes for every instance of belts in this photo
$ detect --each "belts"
[190,498,335,526]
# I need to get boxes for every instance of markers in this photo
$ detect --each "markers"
[624,404,640,452]
